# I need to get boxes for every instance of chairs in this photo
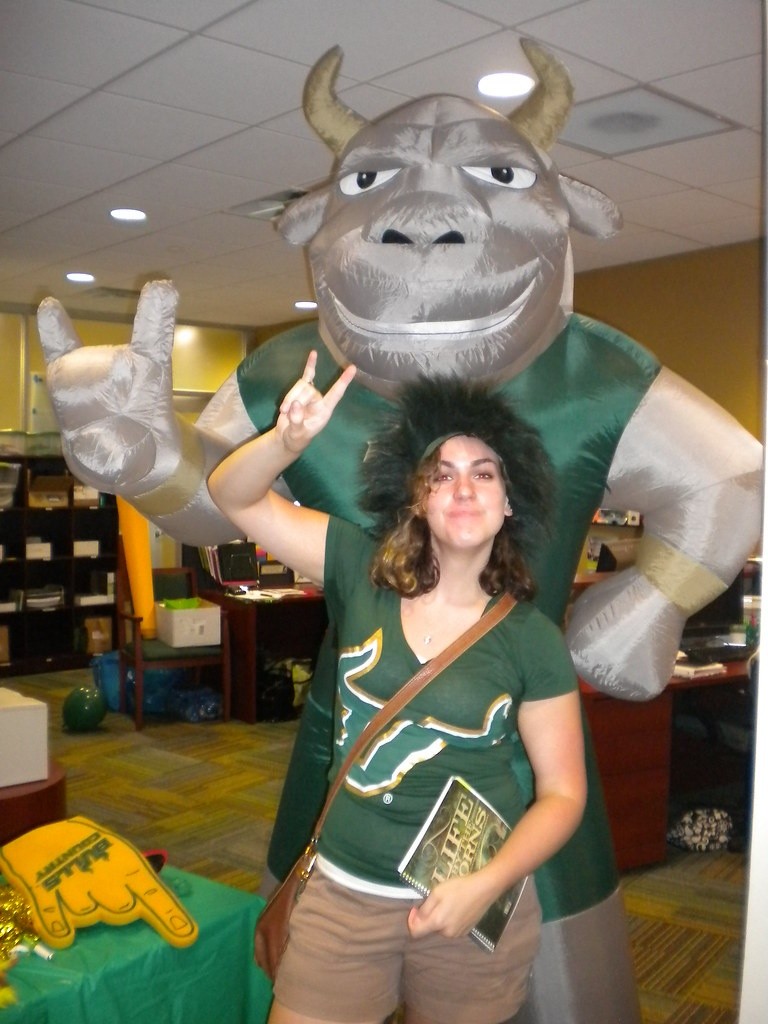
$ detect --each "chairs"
[116,568,231,731]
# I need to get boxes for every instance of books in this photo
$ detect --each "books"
[397,776,529,953]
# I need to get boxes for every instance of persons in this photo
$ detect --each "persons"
[206,349,587,1019]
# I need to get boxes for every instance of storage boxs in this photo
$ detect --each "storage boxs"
[26,537,42,544]
[83,615,113,654]
[0,488,14,512]
[0,461,22,490]
[26,543,53,561]
[0,624,10,664]
[28,432,62,455]
[73,539,100,558]
[28,476,71,508]
[0,429,28,455]
[155,596,221,649]
[626,510,641,526]
[742,595,762,627]
[0,686,48,788]
[74,479,99,509]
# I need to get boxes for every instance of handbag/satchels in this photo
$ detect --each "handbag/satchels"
[253,843,318,982]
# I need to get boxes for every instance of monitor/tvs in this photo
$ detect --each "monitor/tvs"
[682,568,744,638]
[595,538,641,572]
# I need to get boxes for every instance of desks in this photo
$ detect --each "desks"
[202,581,324,723]
[0,863,274,1024]
[573,571,623,604]
[575,659,752,879]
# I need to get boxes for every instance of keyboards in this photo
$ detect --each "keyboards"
[688,645,752,663]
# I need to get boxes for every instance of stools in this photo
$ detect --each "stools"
[0,759,69,847]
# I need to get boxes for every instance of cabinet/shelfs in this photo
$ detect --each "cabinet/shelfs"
[0,455,118,681]
